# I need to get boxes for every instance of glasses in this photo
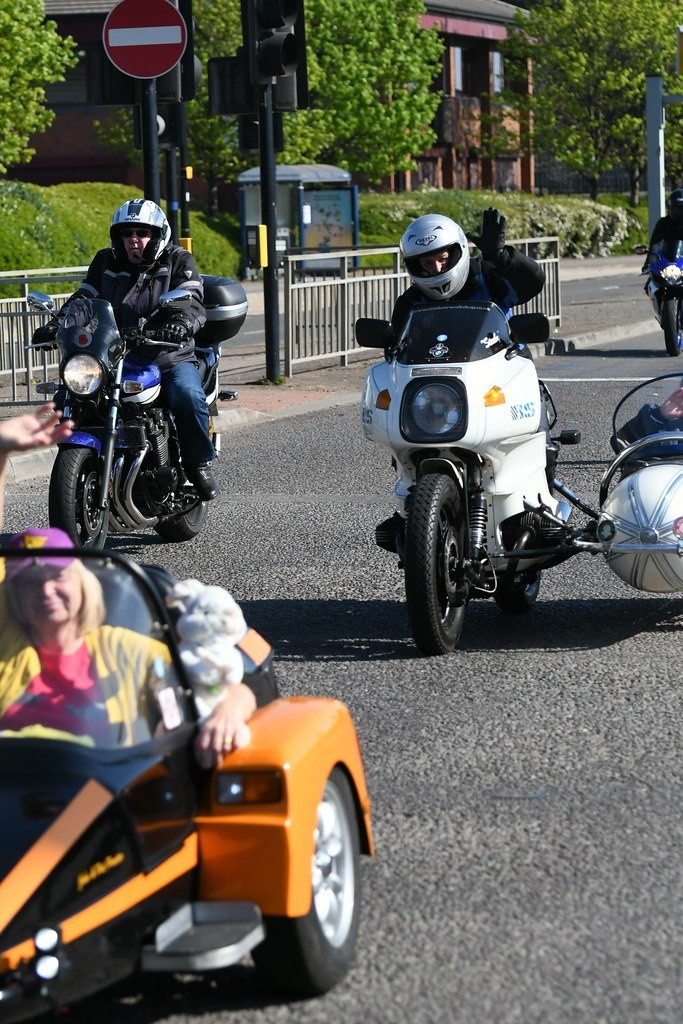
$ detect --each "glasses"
[119,229,150,237]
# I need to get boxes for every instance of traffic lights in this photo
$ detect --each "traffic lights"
[240,0,303,77]
[177,0,203,101]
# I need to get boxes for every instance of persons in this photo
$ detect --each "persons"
[0,402,258,769]
[386,207,553,496]
[31,198,218,500]
[642,189,683,295]
[610,386,683,455]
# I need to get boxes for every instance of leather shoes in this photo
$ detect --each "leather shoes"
[184,467,220,501]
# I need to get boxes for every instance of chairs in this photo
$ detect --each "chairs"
[94,569,155,635]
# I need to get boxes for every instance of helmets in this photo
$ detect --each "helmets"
[665,189,683,218]
[110,199,171,263]
[400,214,470,301]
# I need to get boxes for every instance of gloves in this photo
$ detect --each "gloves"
[154,321,187,344]
[642,263,649,275]
[465,207,506,261]
[32,322,59,352]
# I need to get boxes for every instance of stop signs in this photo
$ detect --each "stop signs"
[102,0,187,78]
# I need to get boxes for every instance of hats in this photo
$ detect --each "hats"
[4,528,75,583]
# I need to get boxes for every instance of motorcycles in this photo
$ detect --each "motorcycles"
[353,303,683,657]
[635,238,683,356]
[26,272,249,552]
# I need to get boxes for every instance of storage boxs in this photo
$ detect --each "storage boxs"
[195,276,250,349]
